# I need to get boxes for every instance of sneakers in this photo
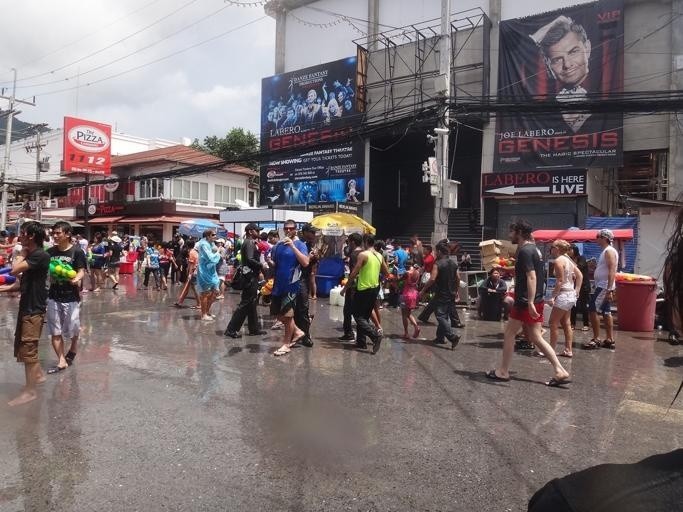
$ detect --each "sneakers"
[224,315,313,347]
[336,317,466,353]
[173,296,224,320]
[88,281,167,291]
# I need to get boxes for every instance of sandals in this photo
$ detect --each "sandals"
[515,325,616,357]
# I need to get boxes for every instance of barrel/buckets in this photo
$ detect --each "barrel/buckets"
[329,285,345,306]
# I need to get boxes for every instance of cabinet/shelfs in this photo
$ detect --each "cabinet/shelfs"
[456,270,489,307]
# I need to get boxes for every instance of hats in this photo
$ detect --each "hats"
[598,229,614,241]
[110,236,123,243]
[245,223,264,230]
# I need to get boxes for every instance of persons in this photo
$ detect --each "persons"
[43,223,90,374]
[224,220,473,356]
[520,19,611,135]
[479,220,618,386]
[525,192,681,510]
[0,220,48,411]
[259,77,360,203]
[0,227,223,321]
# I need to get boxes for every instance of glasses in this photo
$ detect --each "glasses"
[284,227,295,231]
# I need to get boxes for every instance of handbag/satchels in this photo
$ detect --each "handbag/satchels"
[230,264,254,290]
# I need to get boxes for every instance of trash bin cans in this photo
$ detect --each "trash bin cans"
[615,280,657,332]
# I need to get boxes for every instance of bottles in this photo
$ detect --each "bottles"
[284,233,290,245]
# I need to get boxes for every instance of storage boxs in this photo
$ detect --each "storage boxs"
[478,239,503,270]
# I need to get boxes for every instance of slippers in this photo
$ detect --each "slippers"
[484,369,510,382]
[46,366,65,374]
[65,350,76,361]
[545,377,573,386]
[274,346,291,355]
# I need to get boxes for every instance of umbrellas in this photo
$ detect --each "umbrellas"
[178,217,226,241]
[311,211,378,254]
